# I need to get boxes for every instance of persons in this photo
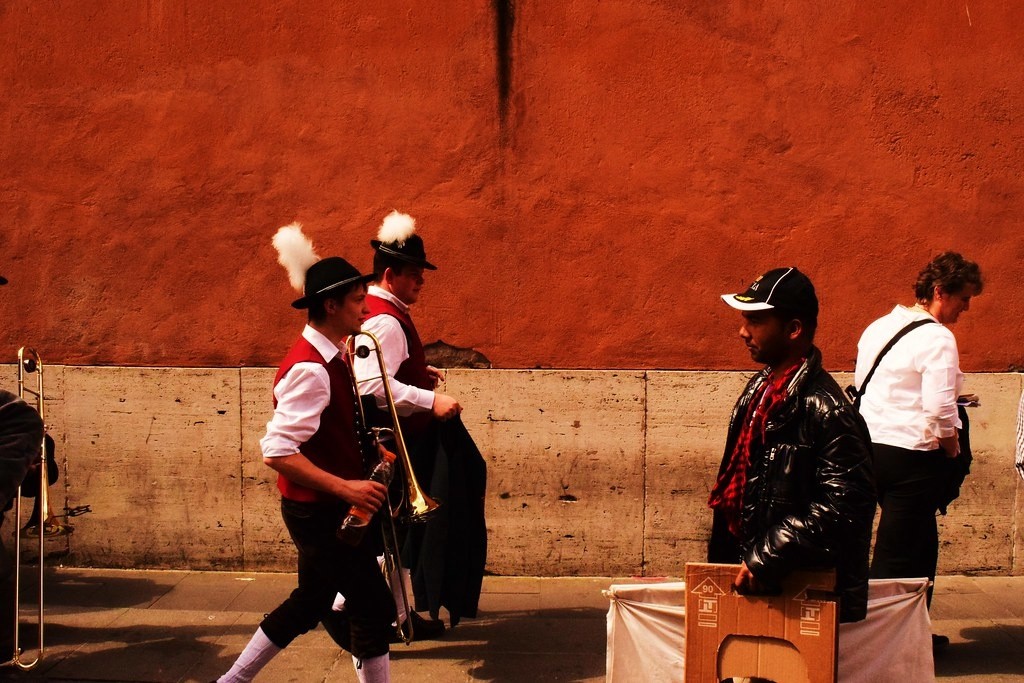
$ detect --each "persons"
[211,222,398,683]
[337,206,487,641]
[853,250,985,648]
[708,267,879,623]
[0,387,60,531]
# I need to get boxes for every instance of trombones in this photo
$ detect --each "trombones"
[347,330,442,646]
[12,345,76,672]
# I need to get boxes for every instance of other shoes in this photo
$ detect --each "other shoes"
[932,633,949,651]
[386,606,447,643]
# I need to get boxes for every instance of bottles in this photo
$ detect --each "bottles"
[344,451,396,526]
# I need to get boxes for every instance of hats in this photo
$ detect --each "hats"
[272,220,378,309]
[719,266,816,311]
[370,210,439,270]
